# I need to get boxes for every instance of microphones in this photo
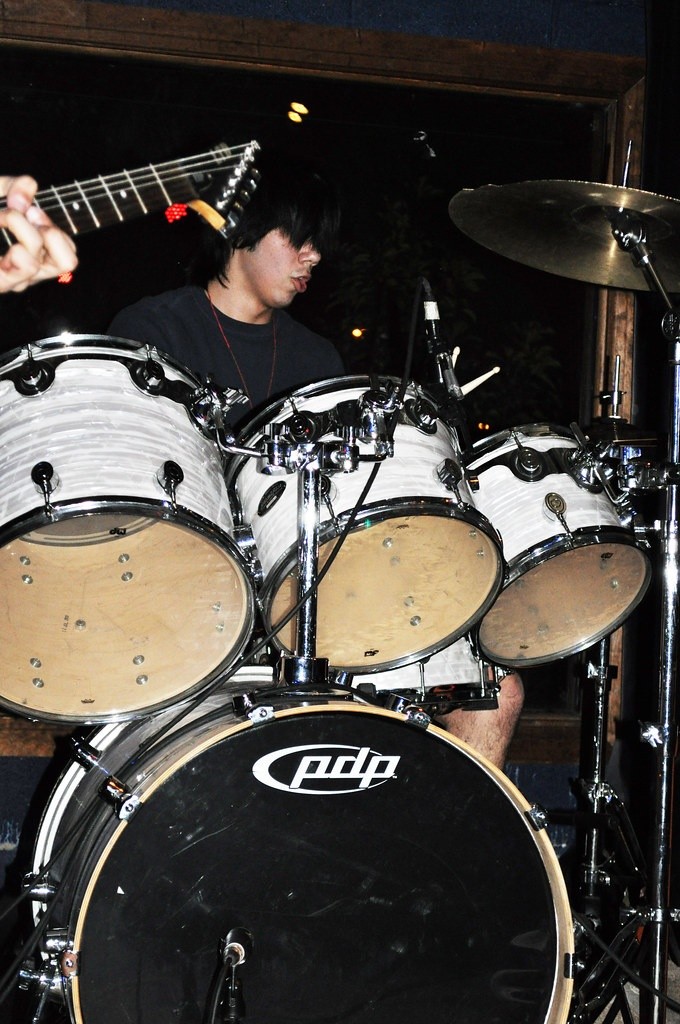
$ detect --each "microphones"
[424,286,447,354]
[222,927,254,964]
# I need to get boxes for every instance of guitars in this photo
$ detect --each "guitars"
[0,134,262,257]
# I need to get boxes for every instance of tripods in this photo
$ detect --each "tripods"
[564,207,680,1024]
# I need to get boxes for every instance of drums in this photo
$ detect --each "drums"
[457,423,653,669]
[26,665,576,1024]
[224,374,505,675]
[0,333,259,726]
[337,627,501,702]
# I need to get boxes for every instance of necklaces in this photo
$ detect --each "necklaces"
[202,289,277,418]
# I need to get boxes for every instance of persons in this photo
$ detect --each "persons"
[0,176,79,294]
[108,163,524,771]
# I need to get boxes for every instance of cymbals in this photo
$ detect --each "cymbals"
[448,179,680,294]
[571,426,658,449]
